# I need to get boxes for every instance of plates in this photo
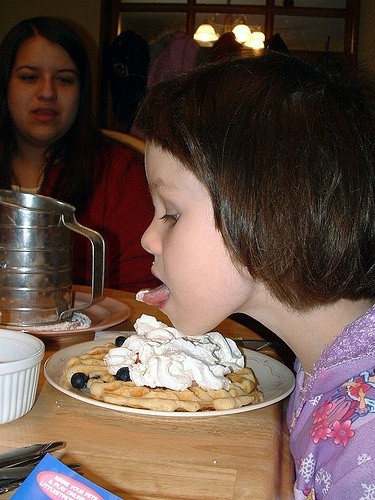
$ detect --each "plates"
[1,290,130,341]
[44,340,295,419]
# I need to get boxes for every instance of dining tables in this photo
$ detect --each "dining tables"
[1,286,295,499]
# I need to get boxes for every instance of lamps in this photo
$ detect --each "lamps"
[194,0,265,49]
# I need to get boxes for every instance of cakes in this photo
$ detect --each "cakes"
[65,312,259,412]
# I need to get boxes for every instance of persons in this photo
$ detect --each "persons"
[0,19,163,294]
[134,51,375,500]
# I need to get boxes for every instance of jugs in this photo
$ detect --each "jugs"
[0,190,105,327]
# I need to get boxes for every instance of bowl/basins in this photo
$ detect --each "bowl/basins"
[0,329,46,425]
